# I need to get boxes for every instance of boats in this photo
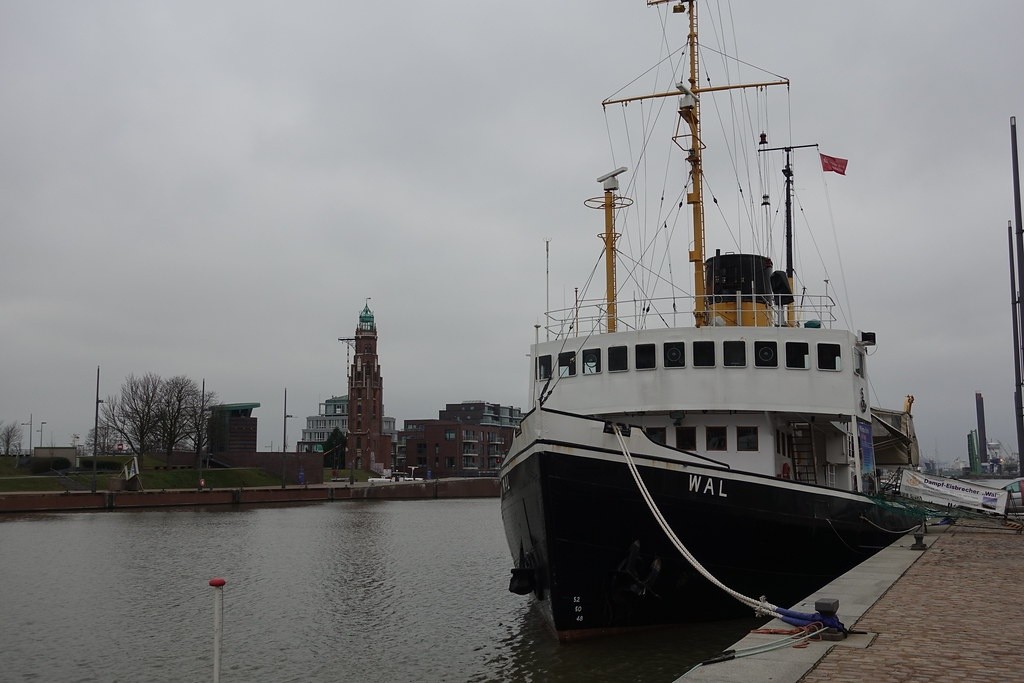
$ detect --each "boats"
[490,0,925,621]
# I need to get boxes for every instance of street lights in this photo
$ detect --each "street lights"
[21,413,32,455]
[281,388,292,487]
[198,378,211,488]
[36,422,46,447]
[92,364,104,492]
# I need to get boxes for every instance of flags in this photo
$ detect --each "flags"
[819,153,848,176]
